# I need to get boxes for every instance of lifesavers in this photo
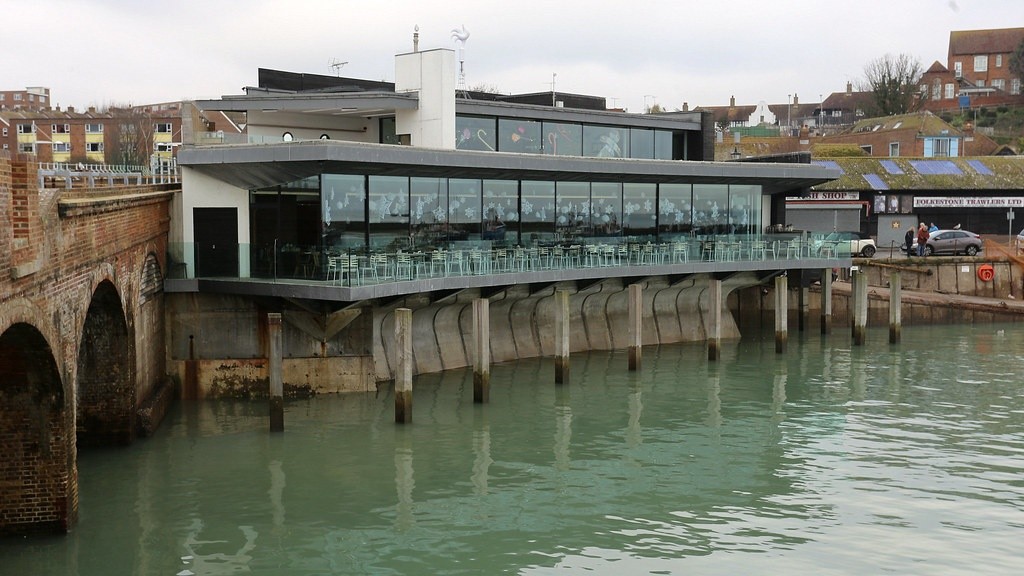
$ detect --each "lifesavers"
[982,270,992,280]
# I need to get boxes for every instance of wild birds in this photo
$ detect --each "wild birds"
[953,223,961,229]
[37,110,39,113]
[997,329,1004,333]
[200,110,203,112]
[22,99,24,101]
[597,131,621,157]
[79,162,84,170]
[1008,294,1015,300]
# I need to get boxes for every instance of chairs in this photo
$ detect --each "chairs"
[327,232,844,287]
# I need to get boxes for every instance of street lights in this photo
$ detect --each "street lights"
[788,95,791,126]
[820,95,823,126]
[552,72,557,108]
[119,131,137,173]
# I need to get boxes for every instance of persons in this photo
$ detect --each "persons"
[904,227,914,257]
[917,222,929,257]
[929,222,939,234]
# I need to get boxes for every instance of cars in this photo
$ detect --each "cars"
[816,230,877,258]
[1015,229,1024,253]
[901,230,983,257]
